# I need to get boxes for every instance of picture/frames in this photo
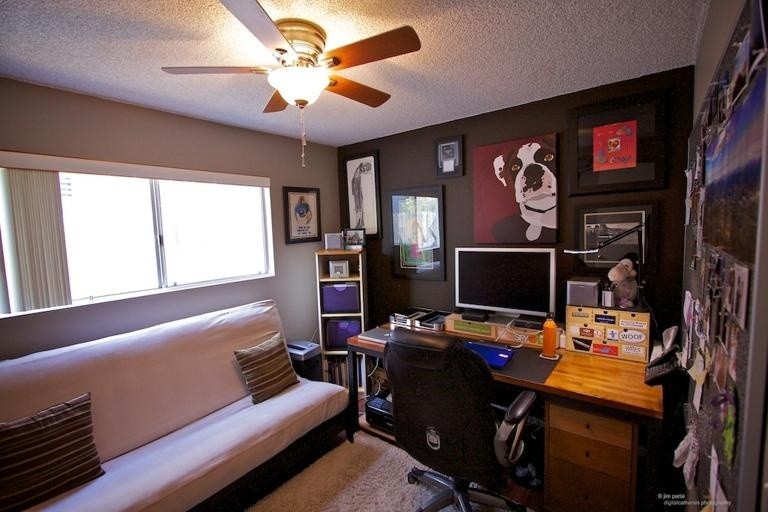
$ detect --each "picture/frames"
[344,229,365,248]
[282,186,321,244]
[343,150,383,241]
[577,204,650,273]
[391,184,447,281]
[564,93,669,196]
[432,134,465,177]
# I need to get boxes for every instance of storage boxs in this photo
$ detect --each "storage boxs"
[326,318,360,348]
[321,283,359,313]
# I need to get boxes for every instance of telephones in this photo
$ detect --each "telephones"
[644,345,689,386]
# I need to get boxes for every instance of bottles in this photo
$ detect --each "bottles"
[543,311,557,358]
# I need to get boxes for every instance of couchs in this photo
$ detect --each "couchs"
[0,299,359,512]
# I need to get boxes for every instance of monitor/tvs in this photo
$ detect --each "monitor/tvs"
[455,247,556,327]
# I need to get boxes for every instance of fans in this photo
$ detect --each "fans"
[161,1,421,113]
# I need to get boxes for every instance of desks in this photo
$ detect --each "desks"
[346,304,664,512]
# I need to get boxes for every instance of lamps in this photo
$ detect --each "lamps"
[563,226,650,313]
[267,62,330,167]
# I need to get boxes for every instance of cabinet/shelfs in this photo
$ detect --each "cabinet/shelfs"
[314,249,369,401]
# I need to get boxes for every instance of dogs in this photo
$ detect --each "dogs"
[491,139,558,242]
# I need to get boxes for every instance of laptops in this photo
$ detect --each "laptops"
[358,328,393,345]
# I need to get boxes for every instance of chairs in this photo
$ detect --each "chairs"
[381,325,542,512]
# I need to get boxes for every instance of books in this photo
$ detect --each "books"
[324,357,362,389]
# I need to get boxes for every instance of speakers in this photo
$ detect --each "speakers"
[567,275,602,308]
[324,233,342,250]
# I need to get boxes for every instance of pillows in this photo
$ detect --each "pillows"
[233,329,300,404]
[0,390,106,512]
